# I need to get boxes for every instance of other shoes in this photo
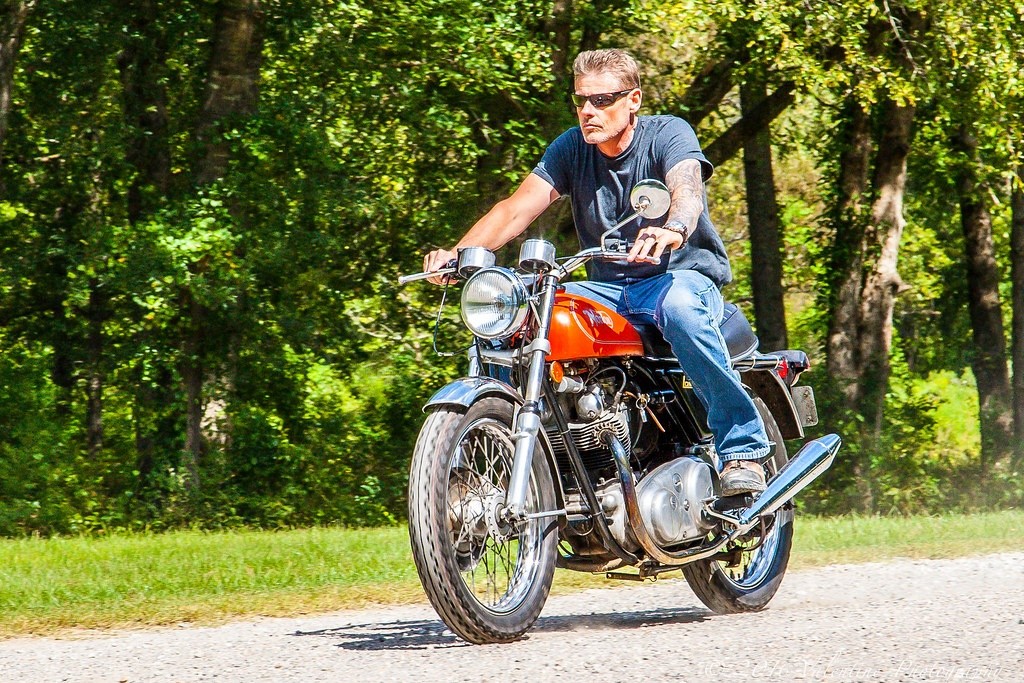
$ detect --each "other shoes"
[718,459,768,497]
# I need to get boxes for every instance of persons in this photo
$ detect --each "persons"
[420,48,778,497]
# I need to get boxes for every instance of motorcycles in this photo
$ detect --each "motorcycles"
[407,179,843,644]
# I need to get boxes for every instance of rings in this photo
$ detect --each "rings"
[638,233,649,240]
[646,233,656,240]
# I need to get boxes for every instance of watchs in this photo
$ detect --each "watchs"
[661,220,689,251]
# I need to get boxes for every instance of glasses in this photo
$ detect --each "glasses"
[571,88,634,108]
